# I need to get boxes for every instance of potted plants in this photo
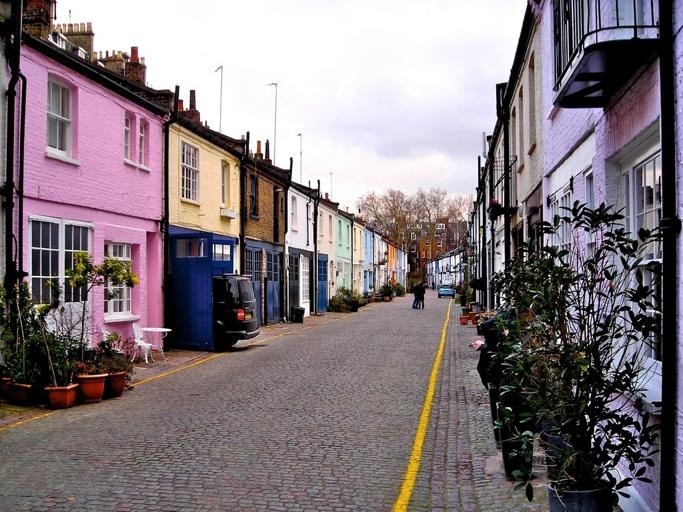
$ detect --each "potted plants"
[0,246,139,410]
[325,277,407,313]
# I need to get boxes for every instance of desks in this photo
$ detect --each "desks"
[138,325,173,361]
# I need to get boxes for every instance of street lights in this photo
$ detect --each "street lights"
[328,172,333,200]
[296,132,302,184]
[266,83,277,166]
[213,66,222,131]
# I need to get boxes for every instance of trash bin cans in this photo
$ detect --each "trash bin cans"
[295,308,305,323]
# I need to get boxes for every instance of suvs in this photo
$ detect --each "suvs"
[211,271,259,350]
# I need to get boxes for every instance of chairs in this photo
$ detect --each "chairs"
[128,318,155,365]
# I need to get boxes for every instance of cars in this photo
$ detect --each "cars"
[437,285,455,298]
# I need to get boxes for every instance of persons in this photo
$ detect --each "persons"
[410,283,419,309]
[417,282,425,309]
